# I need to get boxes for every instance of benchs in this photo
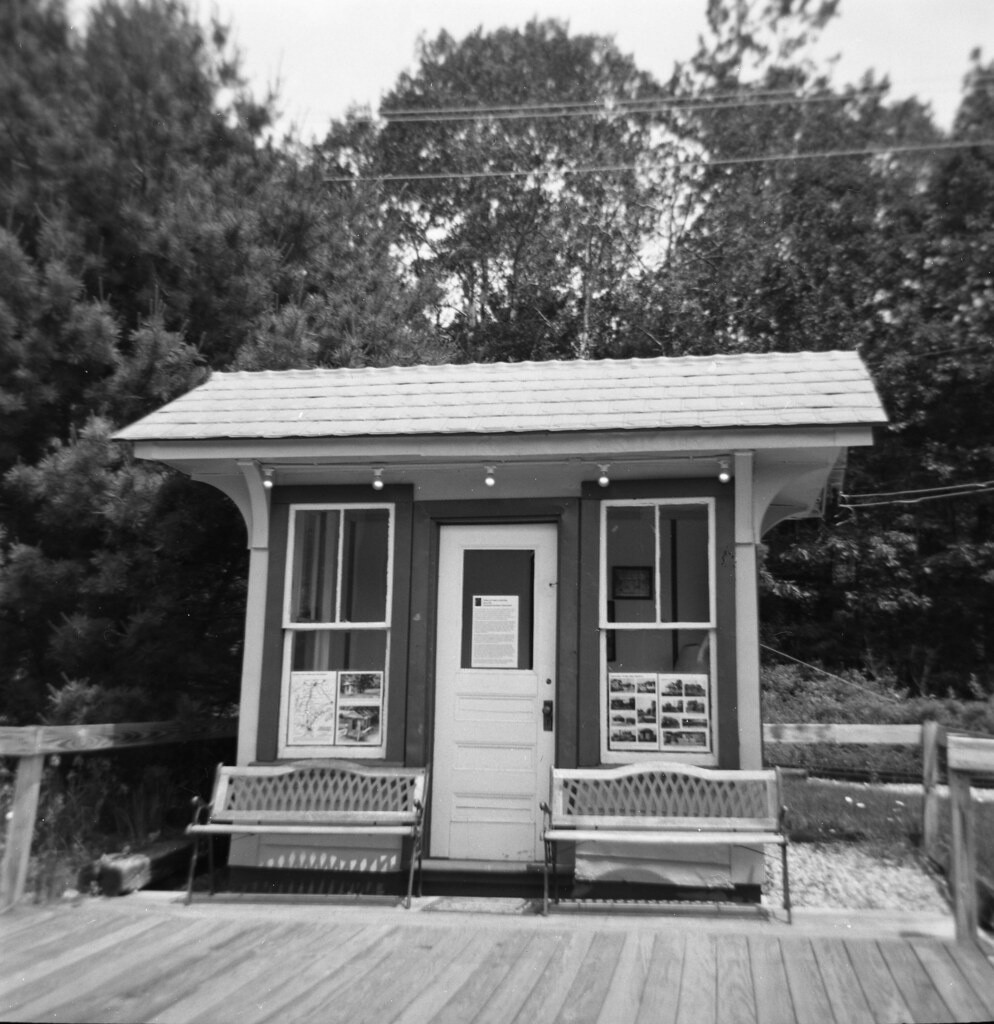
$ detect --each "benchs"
[183,759,432,910]
[538,761,794,925]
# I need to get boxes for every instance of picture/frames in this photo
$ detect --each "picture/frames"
[612,565,654,600]
